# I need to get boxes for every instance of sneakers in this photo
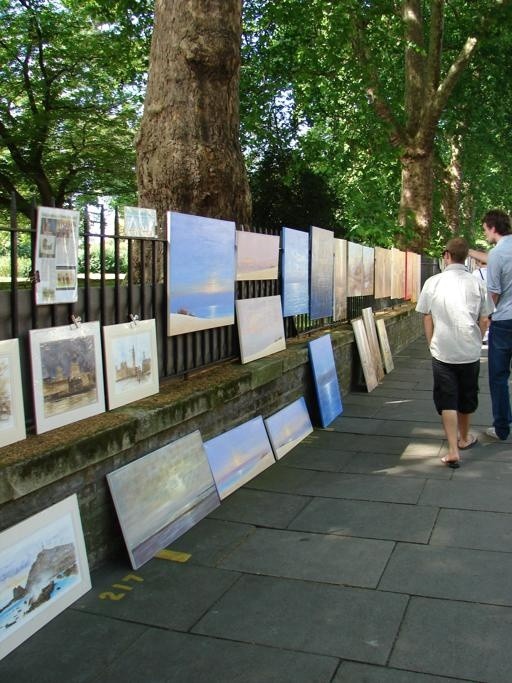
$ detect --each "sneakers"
[486,427,500,438]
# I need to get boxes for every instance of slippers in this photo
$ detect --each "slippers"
[441,456,460,467]
[459,433,478,449]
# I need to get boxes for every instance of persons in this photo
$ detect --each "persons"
[473,251,496,345]
[415,237,488,469]
[467,209,512,441]
